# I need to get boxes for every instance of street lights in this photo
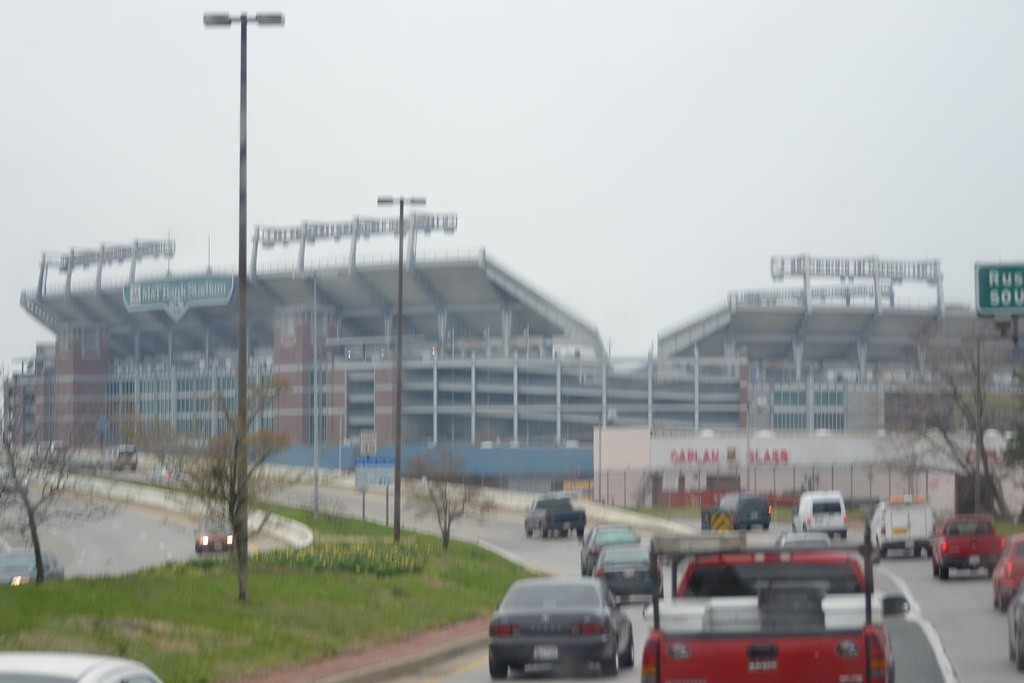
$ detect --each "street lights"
[377,196,425,538]
[201,11,286,602]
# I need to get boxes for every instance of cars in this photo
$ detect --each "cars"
[775,534,831,546]
[194,519,235,556]
[486,578,633,681]
[991,533,1024,614]
[109,445,137,472]
[1006,580,1024,674]
[0,549,64,587]
[578,524,642,577]
[590,545,662,602]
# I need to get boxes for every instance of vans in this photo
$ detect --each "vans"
[719,492,772,532]
[793,490,848,541]
[30,441,67,465]
[864,494,937,558]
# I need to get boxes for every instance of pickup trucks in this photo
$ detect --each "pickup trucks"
[931,515,1007,581]
[639,545,910,682]
[523,497,589,539]
